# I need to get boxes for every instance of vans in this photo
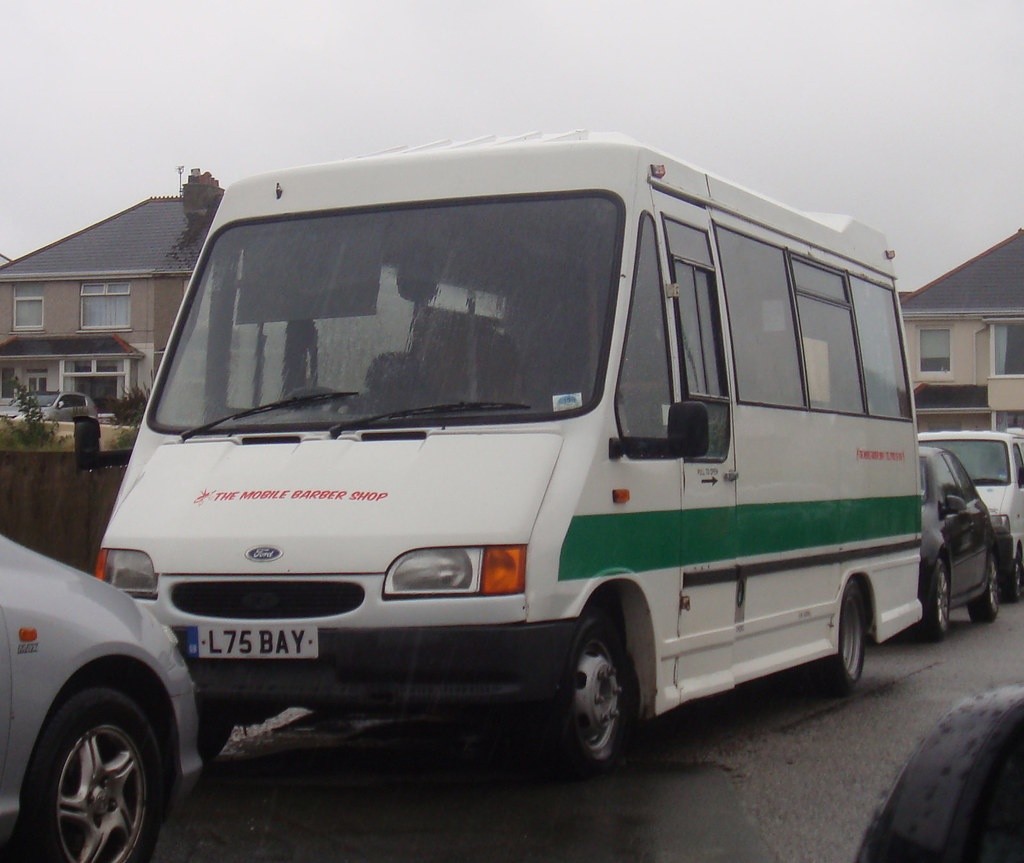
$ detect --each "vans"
[913,428,1024,603]
[96,132,928,781]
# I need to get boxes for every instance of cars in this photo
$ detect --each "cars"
[0,534,202,863]
[0,391,98,423]
[916,447,1003,640]
